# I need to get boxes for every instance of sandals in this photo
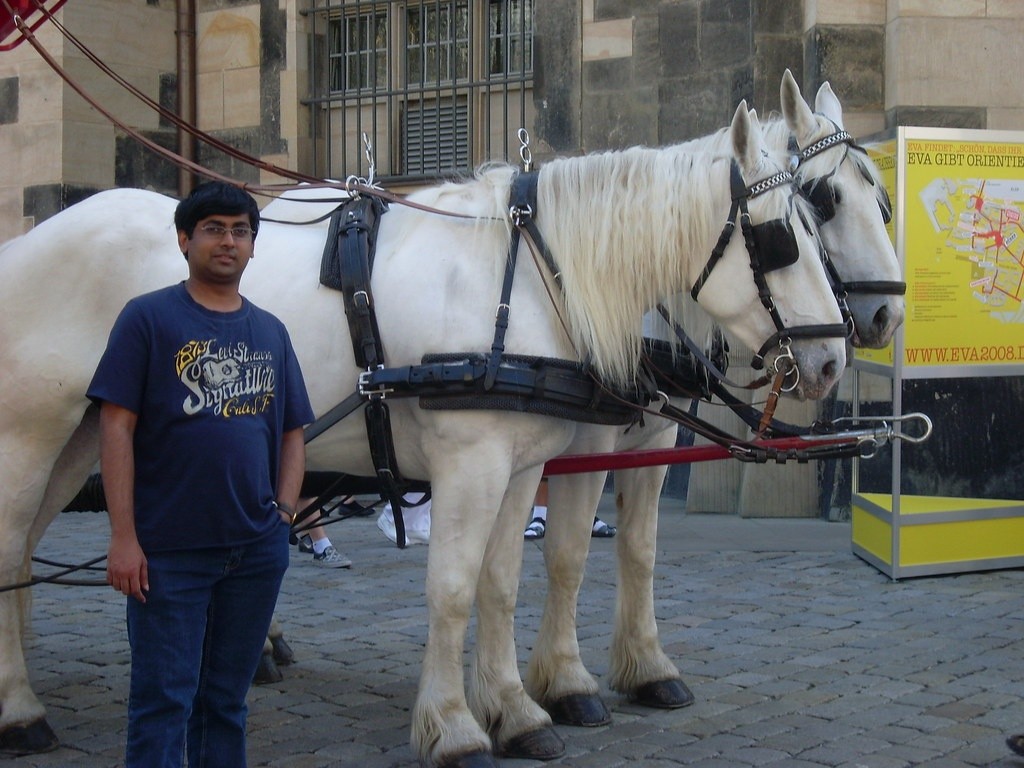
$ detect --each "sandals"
[524,517,545,539]
[591,516,616,538]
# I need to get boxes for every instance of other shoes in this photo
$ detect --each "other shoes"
[313,546,352,567]
[298,538,314,553]
[1006,735,1024,755]
[338,500,376,517]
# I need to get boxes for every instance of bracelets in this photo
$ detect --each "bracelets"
[277,503,297,526]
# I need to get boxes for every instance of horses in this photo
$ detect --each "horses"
[0,96,856,768]
[241,64,911,692]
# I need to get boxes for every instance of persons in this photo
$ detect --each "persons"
[524,481,617,540]
[376,492,433,545]
[298,494,374,567]
[85,181,314,768]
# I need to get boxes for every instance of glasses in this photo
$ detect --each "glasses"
[190,225,257,240]
